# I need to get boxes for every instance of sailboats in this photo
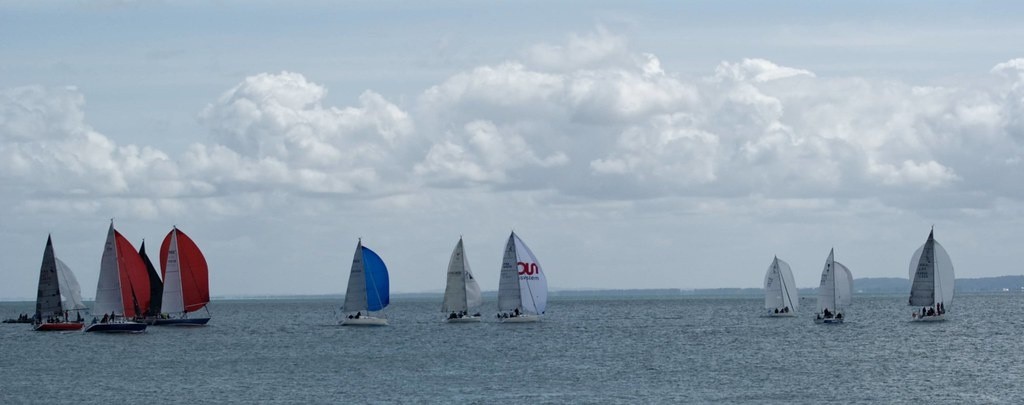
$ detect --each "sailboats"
[136,226,212,326]
[84,219,146,333]
[32,233,85,331]
[761,255,803,317]
[814,248,854,325]
[337,237,390,326]
[441,236,484,323]
[906,226,955,321]
[493,231,549,323]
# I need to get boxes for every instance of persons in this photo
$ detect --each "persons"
[515,308,519,315]
[19,313,27,320]
[775,308,778,313]
[780,306,789,313]
[55,316,60,322]
[92,313,109,324]
[65,310,69,322]
[348,312,361,319]
[817,308,841,320]
[47,316,50,322]
[156,312,167,318]
[474,312,481,317]
[497,313,508,318]
[458,311,463,318]
[450,311,457,318]
[509,309,514,317]
[921,302,945,317]
[109,311,115,322]
